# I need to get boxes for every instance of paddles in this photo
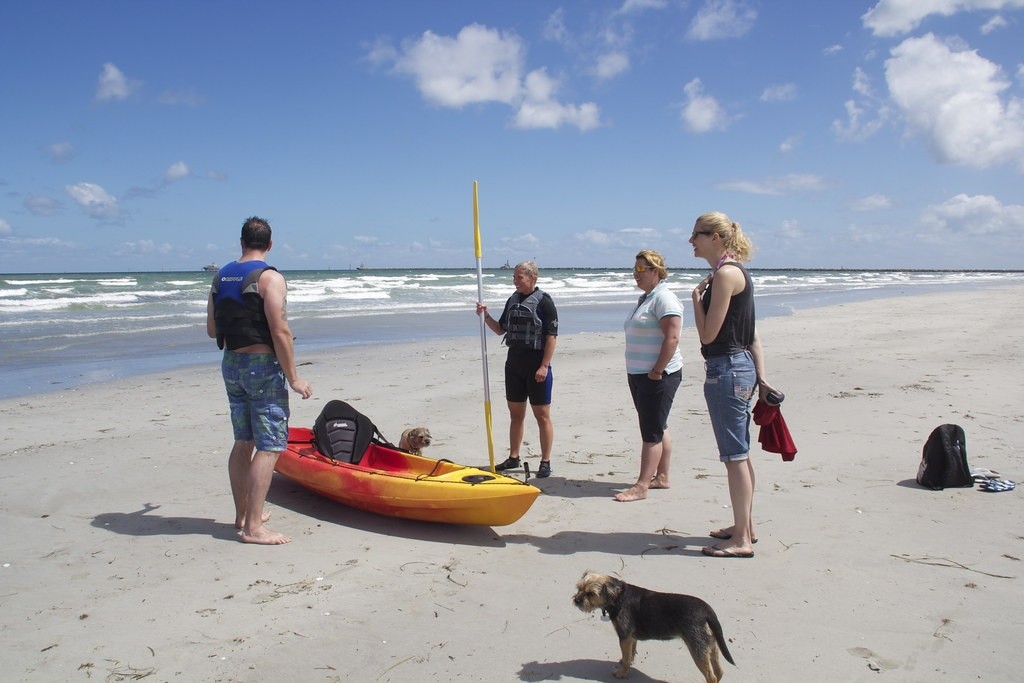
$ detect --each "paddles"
[472,180,495,474]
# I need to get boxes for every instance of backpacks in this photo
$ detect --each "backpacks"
[917,424,975,491]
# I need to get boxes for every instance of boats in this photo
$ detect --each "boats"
[203,262,220,272]
[274,426,542,527]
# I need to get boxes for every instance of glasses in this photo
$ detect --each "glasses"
[633,266,653,273]
[692,231,725,239]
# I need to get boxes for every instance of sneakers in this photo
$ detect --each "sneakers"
[537,460,551,478]
[495,456,522,471]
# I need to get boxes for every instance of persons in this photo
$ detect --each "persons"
[475,260,558,477]
[688,212,781,558]
[207,214,314,545]
[613,251,684,502]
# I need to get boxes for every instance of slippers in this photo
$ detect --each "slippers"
[710,529,759,543]
[702,544,754,558]
[970,468,1000,482]
[979,478,1015,491]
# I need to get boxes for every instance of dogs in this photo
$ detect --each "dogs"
[573,570,736,683]
[399,427,433,457]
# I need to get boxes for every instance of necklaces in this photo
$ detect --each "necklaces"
[713,253,728,278]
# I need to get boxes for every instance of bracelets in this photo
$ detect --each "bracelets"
[651,368,662,375]
[484,314,489,318]
